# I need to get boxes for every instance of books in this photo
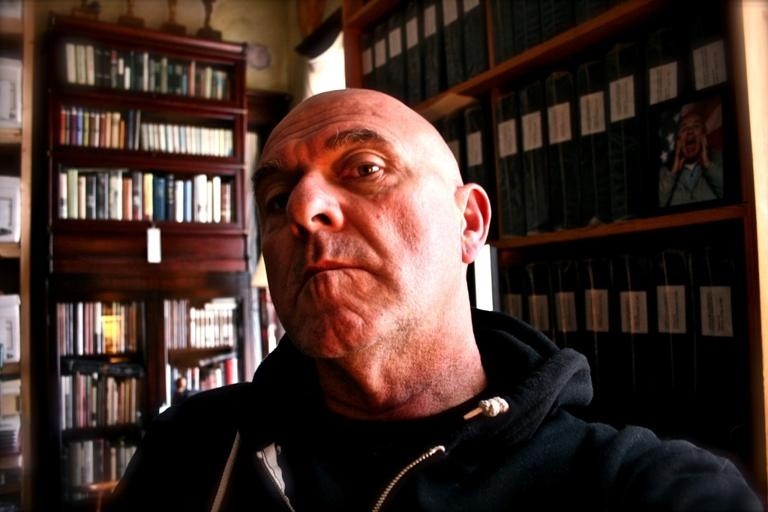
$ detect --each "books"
[58,106,142,150]
[62,439,137,487]
[145,122,234,158]
[164,352,239,406]
[358,1,489,107]
[163,297,238,349]
[65,40,234,101]
[58,168,232,224]
[57,301,146,357]
[60,372,137,430]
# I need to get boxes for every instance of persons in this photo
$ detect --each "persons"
[659,105,720,205]
[100,89,763,512]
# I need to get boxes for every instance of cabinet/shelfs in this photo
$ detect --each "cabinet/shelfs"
[32,9,257,509]
[0,3,31,512]
[341,1,768,509]
